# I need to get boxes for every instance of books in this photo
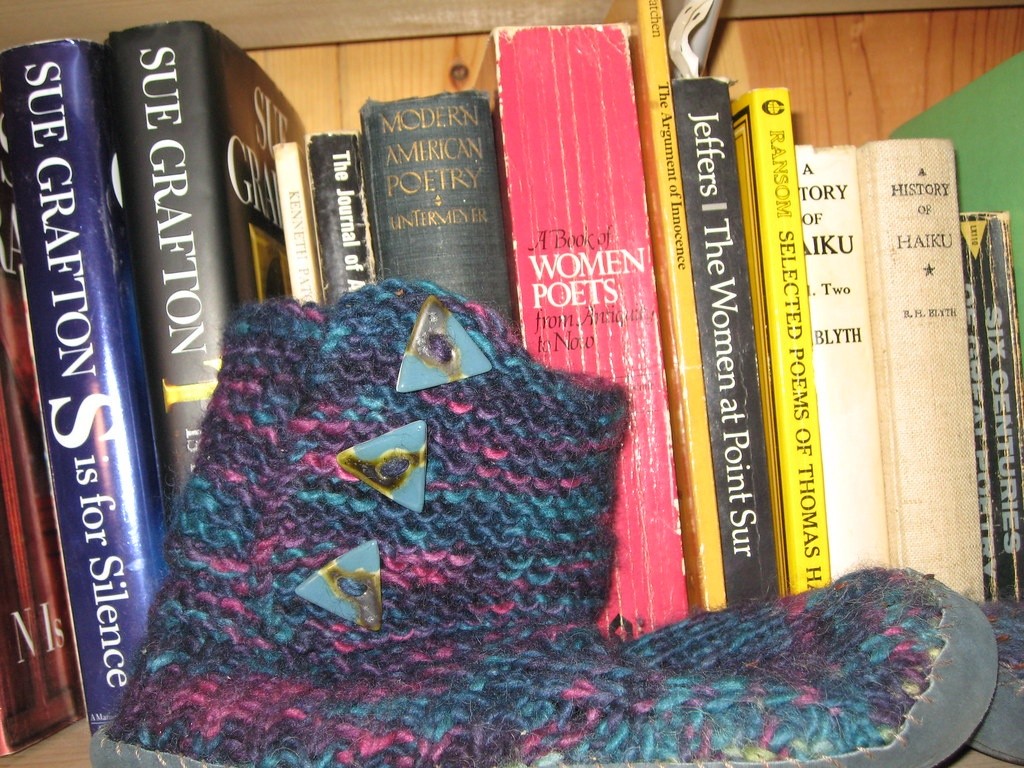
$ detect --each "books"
[271,88,516,336]
[670,73,831,617]
[0,22,307,757]
[794,86,1024,606]
[491,0,726,645]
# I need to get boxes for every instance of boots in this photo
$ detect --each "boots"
[958,596,1024,765]
[85,280,999,767]
